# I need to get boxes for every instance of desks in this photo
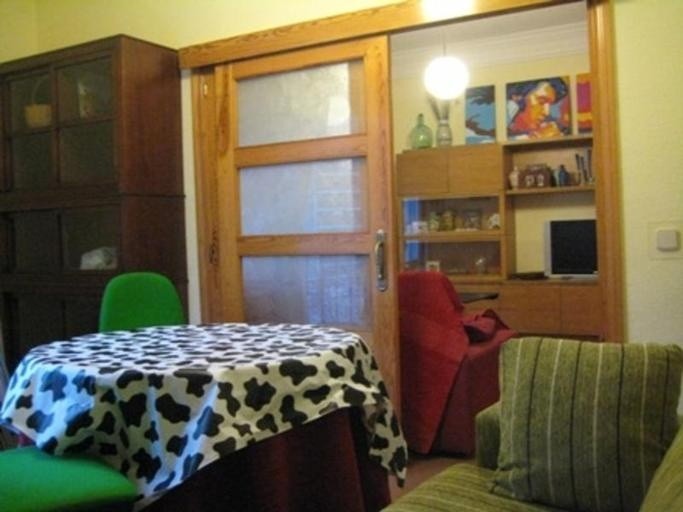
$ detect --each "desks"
[23,325,387,510]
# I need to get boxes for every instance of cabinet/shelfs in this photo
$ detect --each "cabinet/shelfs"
[380,126,614,339]
[4,34,186,327]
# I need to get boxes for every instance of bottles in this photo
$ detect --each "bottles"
[409,112,433,148]
[508,164,570,189]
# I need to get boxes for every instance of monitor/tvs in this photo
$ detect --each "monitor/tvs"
[542,218,598,278]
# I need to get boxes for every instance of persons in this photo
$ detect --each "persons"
[506,77,569,140]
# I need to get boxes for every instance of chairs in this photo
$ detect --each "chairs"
[95,267,187,335]
[387,359,683,511]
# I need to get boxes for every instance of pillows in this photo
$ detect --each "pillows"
[484,334,683,506]
